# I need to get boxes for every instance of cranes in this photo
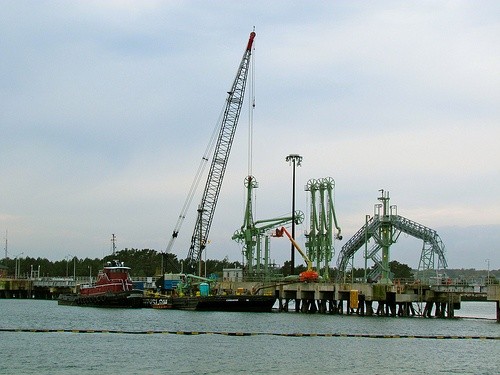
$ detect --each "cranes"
[110,233,118,254]
[231,173,306,273]
[336,187,449,281]
[161,25,257,276]
[304,177,344,282]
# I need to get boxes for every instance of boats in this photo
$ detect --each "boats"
[197,294,278,312]
[57,259,143,308]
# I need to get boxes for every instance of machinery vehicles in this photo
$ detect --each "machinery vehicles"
[270,226,319,284]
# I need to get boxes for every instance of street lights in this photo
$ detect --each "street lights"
[15,252,24,279]
[485,257,490,284]
